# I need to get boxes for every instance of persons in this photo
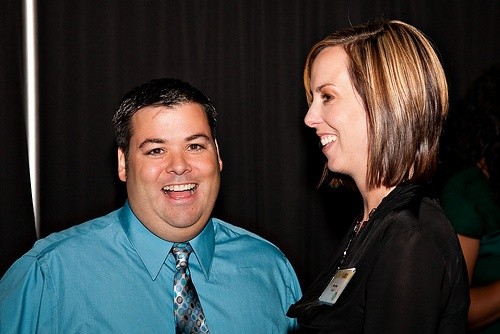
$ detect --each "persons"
[439,64,500,334]
[1,78,305,334]
[286,19,472,334]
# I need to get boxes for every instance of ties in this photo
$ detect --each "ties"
[170,241,209,334]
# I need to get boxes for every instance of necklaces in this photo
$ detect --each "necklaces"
[336,208,377,270]
